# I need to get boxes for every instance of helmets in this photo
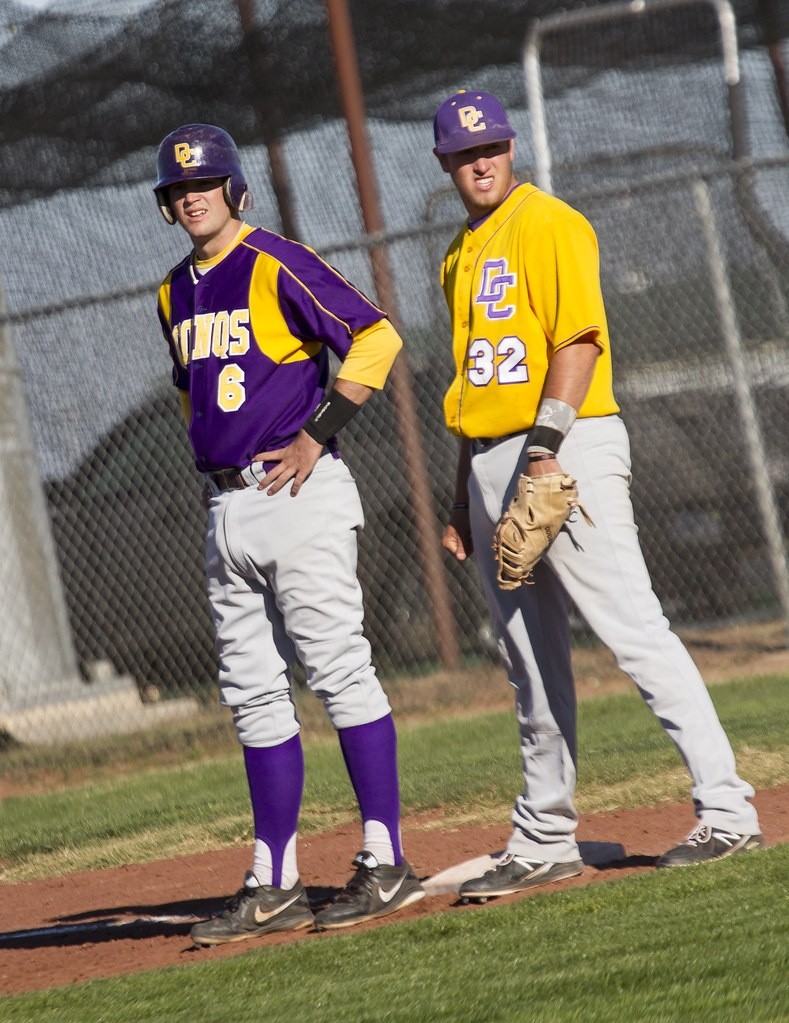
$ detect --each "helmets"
[152,123,255,226]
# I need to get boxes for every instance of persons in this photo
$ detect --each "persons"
[432,90,762,903]
[153,123,428,944]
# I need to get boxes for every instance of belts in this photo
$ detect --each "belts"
[473,426,532,451]
[202,435,340,495]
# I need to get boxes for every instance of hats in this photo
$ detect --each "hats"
[432,90,517,157]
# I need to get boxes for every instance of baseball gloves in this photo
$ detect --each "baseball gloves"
[490,470,596,593]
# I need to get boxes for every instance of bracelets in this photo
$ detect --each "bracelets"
[303,388,363,446]
[526,398,578,462]
[451,502,470,510]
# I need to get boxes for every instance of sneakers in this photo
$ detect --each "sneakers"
[459,848,585,904]
[189,871,315,946]
[655,823,765,868]
[314,849,426,930]
